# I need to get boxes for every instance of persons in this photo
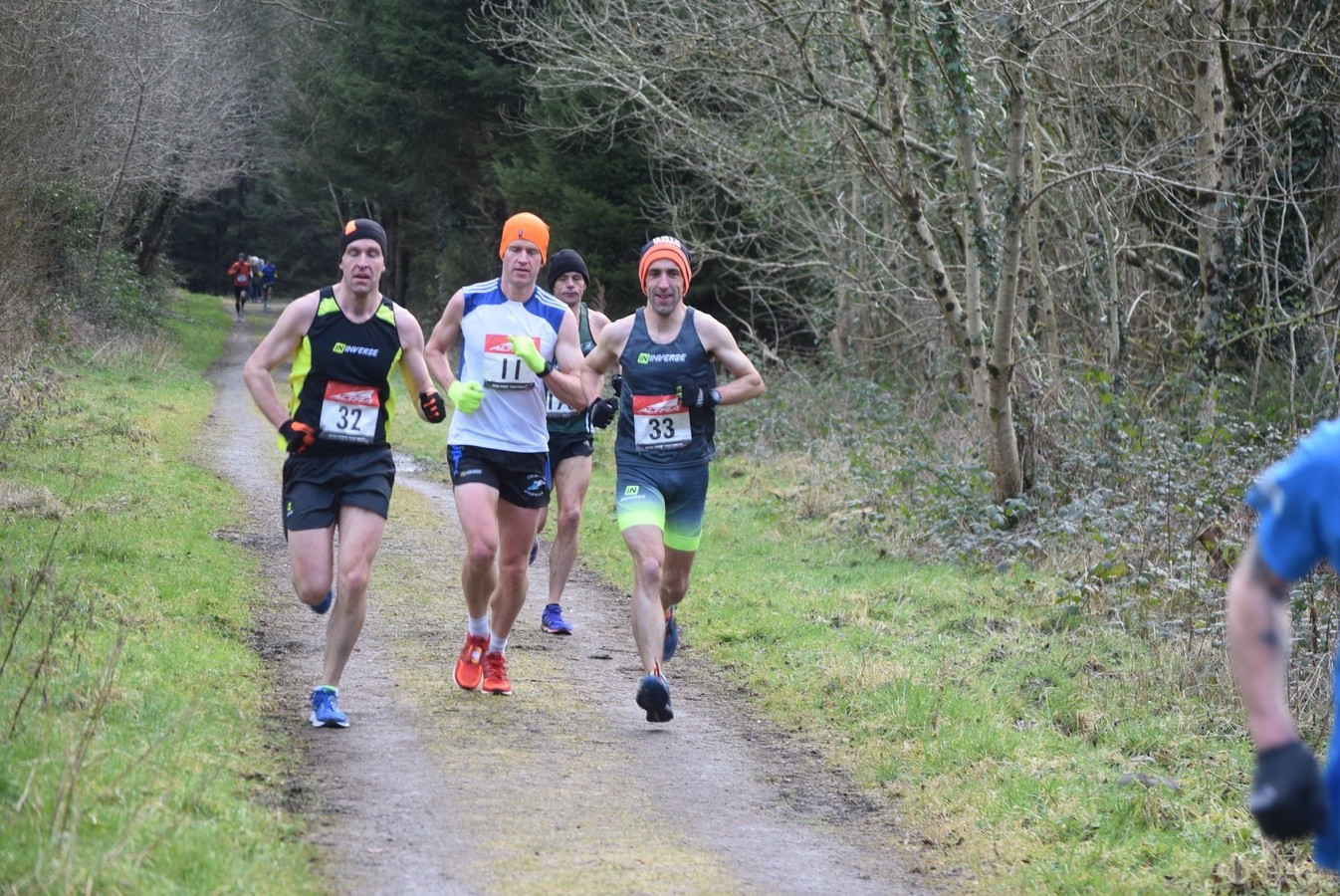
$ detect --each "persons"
[527,249,624,634]
[246,218,445,730]
[247,255,277,314]
[1223,415,1340,876]
[229,253,251,322]
[581,236,762,723]
[423,212,586,695]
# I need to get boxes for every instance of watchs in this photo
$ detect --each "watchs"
[534,361,553,378]
[705,388,721,408]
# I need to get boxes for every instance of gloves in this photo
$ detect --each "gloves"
[1249,741,1330,837]
[447,379,485,414]
[611,374,623,398]
[276,420,318,455]
[507,334,546,375]
[418,387,445,423]
[587,396,618,430]
[676,383,722,410]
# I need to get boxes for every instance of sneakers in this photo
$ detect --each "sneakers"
[636,657,673,721]
[529,535,540,563]
[453,628,491,692]
[481,649,513,697]
[309,687,351,728]
[313,589,333,614]
[542,604,573,634]
[663,602,678,660]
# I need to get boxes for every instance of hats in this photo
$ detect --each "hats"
[545,249,590,294]
[341,218,386,265]
[638,236,692,299]
[499,211,550,268]
